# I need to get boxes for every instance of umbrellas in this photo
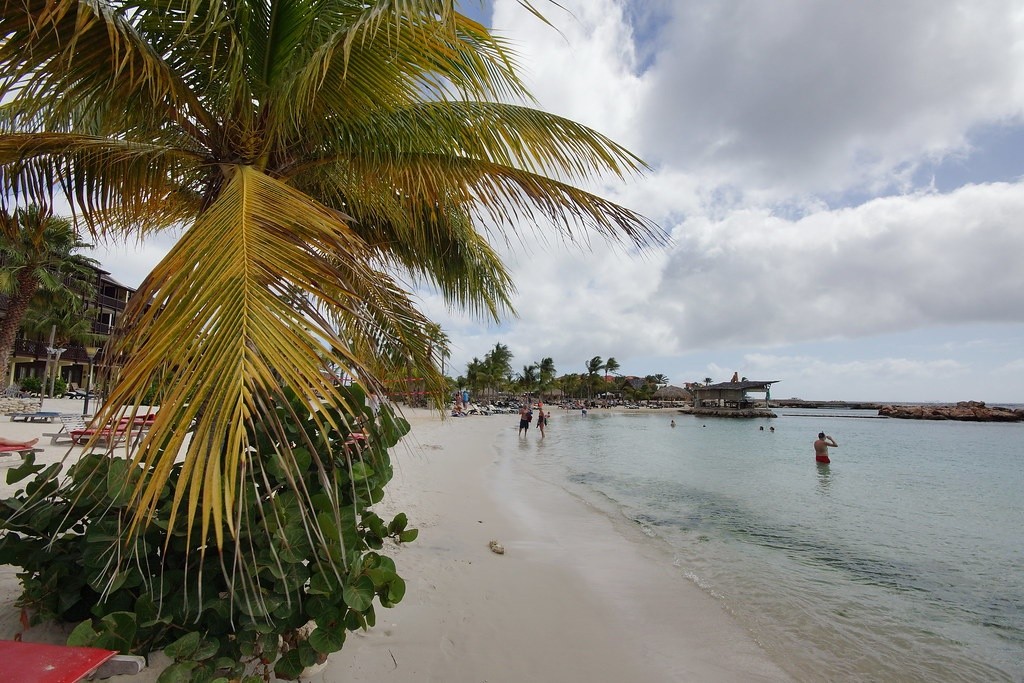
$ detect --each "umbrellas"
[602,392,614,397]
[544,388,567,401]
[653,385,691,405]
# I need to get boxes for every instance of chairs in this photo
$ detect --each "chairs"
[60,382,99,399]
[5,412,155,445]
[466,403,518,416]
[0,444,45,460]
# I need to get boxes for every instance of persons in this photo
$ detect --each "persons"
[538,407,545,438]
[452,391,468,417]
[544,412,551,426]
[671,420,676,427]
[760,426,763,430]
[582,408,587,418]
[769,426,774,431]
[814,432,838,463]
[518,405,532,437]
